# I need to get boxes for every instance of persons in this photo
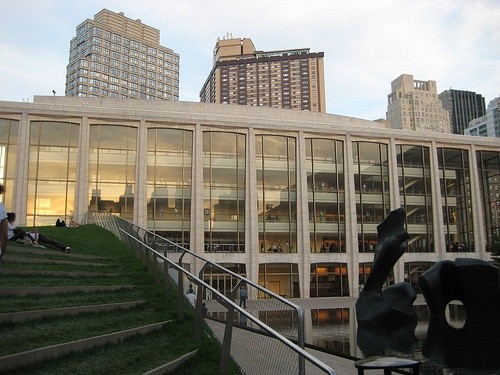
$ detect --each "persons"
[0,184,72,255]
[186,284,196,307]
[451,241,467,252]
[328,242,373,252]
[261,240,290,252]
[239,286,248,308]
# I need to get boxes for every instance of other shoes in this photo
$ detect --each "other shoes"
[65,246,72,253]
